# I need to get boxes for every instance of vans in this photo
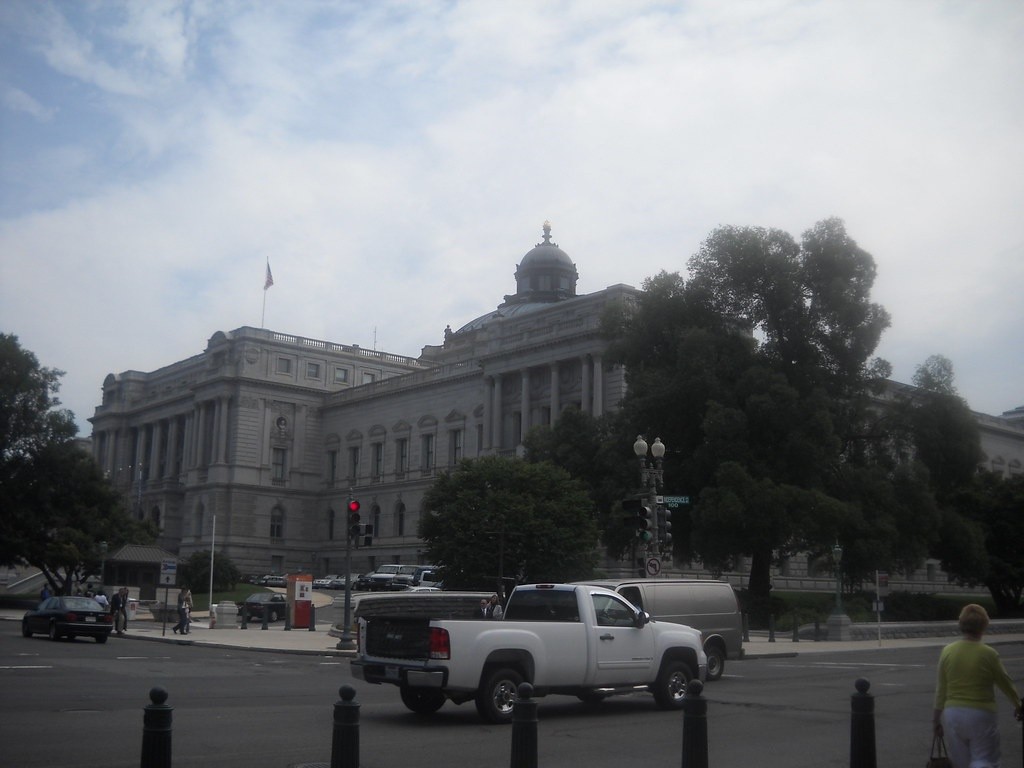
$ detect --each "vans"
[369,565,404,592]
[248,574,289,589]
[563,580,748,682]
[412,567,444,587]
[393,564,439,590]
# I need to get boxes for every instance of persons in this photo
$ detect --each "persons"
[41,583,49,602]
[932,604,1024,768]
[83,583,129,634]
[476,595,503,620]
[173,584,193,635]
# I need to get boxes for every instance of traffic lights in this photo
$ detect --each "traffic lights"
[659,506,674,547]
[639,505,656,546]
[359,523,374,549]
[347,499,361,537]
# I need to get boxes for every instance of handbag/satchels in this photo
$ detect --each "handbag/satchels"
[926,734,952,768]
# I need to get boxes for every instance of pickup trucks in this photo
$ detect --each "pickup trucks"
[349,581,708,726]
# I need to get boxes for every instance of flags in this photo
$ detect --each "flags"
[264,264,273,290]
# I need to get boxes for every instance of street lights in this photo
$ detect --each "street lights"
[829,539,855,642]
[634,435,670,582]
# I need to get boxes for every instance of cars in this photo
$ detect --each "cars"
[242,592,287,623]
[357,571,377,589]
[313,573,340,588]
[22,595,115,642]
[401,588,442,592]
[333,573,363,591]
